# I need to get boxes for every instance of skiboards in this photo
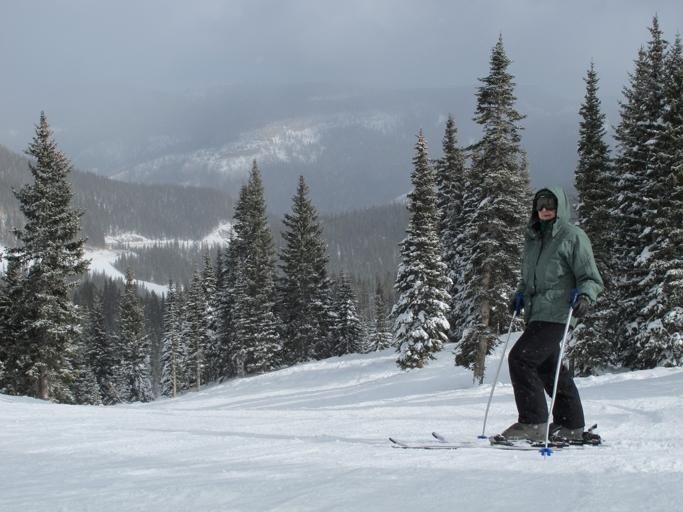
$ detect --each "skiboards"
[388,429,602,451]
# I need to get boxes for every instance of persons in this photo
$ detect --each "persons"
[491,186,605,442]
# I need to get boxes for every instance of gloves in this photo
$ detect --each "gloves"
[508,293,523,315]
[572,297,588,318]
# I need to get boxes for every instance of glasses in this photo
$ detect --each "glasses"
[534,195,556,211]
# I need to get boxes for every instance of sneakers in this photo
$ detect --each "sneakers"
[501,422,549,443]
[549,424,584,440]
